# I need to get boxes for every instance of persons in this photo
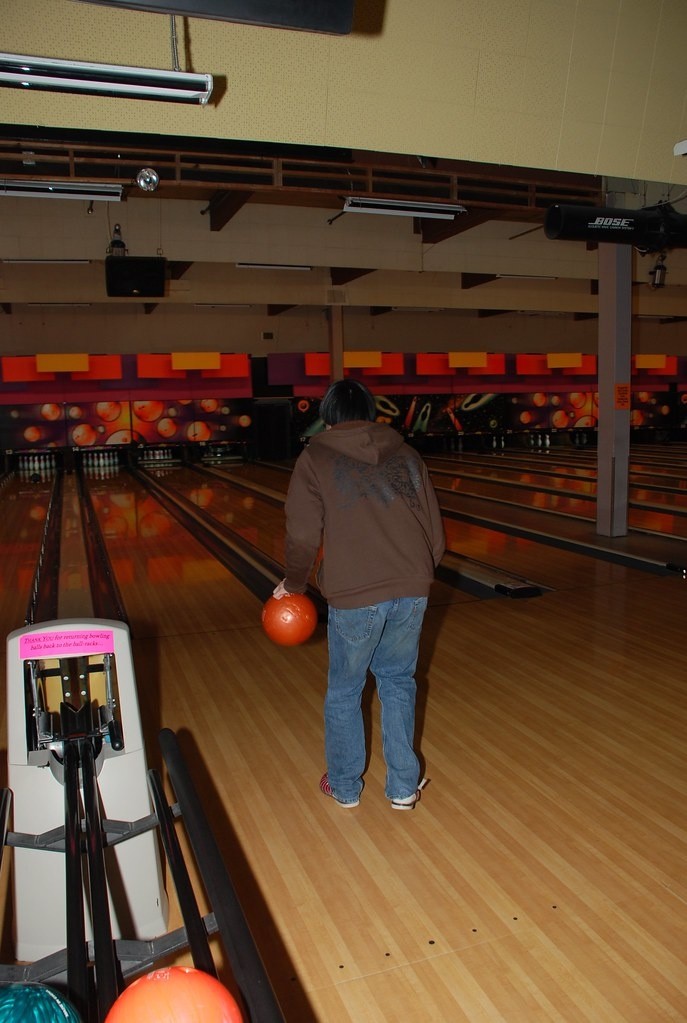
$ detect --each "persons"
[272,377,447,812]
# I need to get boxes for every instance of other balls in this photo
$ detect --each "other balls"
[1,979,79,1022]
[104,965,243,1023]
[262,594,317,645]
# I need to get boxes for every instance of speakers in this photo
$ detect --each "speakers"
[105,256,165,298]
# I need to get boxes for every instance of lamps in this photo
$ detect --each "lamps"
[105,199,166,298]
[0,179,123,202]
[0,14,214,106]
[235,263,312,271]
[496,274,558,280]
[194,304,252,308]
[327,195,467,225]
[136,167,160,192]
[28,303,91,307]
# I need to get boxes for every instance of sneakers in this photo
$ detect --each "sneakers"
[320,772,360,808]
[390,792,417,810]
